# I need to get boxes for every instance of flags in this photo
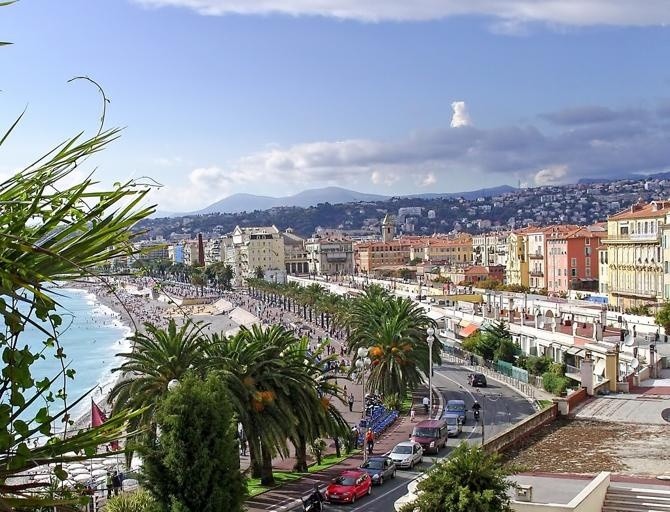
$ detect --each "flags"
[91,398,122,451]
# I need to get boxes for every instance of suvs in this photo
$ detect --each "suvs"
[467,373,487,387]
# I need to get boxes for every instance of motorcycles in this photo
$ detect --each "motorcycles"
[473,407,481,421]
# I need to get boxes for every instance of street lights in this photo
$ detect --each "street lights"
[425,327,436,418]
[355,346,371,464]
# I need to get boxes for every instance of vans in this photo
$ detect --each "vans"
[409,419,448,454]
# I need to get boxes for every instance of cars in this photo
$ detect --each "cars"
[358,456,397,486]
[443,399,468,424]
[325,469,372,503]
[389,440,424,471]
[440,413,465,438]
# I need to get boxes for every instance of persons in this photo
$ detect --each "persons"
[348,392,355,411]
[411,404,415,421]
[106,472,113,498]
[118,472,124,490]
[93,270,238,328]
[423,395,430,413]
[366,427,375,455]
[352,425,360,448]
[343,384,348,401]
[112,470,120,495]
[308,490,324,511]
[238,295,362,382]
[240,438,248,456]
[472,400,481,410]
